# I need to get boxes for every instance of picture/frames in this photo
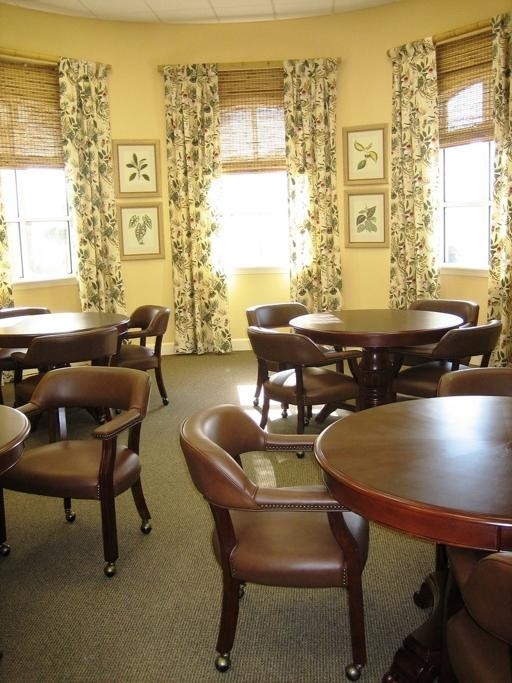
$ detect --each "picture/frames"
[344,188,390,248]
[342,123,389,185]
[117,203,165,260]
[112,139,161,197]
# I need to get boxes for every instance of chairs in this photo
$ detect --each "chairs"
[412,366,512,400]
[247,327,365,457]
[2,306,50,318]
[110,305,170,406]
[12,326,119,425]
[397,319,500,397]
[392,301,479,367]
[381,552,510,680]
[178,404,371,666]
[2,366,151,576]
[247,302,330,418]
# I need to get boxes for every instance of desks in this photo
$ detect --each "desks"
[2,403,31,453]
[286,309,463,398]
[1,312,129,370]
[316,395,512,613]
